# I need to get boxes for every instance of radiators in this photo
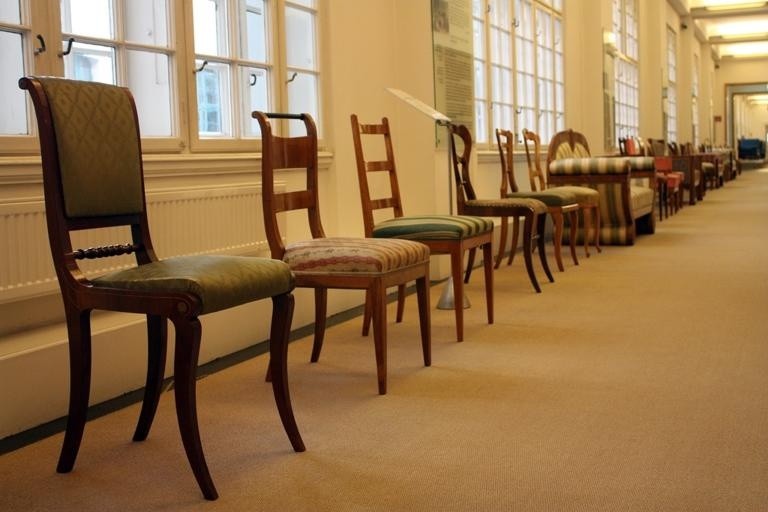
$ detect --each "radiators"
[1,178,289,307]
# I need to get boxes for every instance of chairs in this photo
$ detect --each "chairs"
[448,121,556,294]
[546,129,657,246]
[349,113,496,343]
[15,74,308,500]
[251,109,434,396]
[617,136,767,221]
[494,127,581,273]
[523,128,604,259]
